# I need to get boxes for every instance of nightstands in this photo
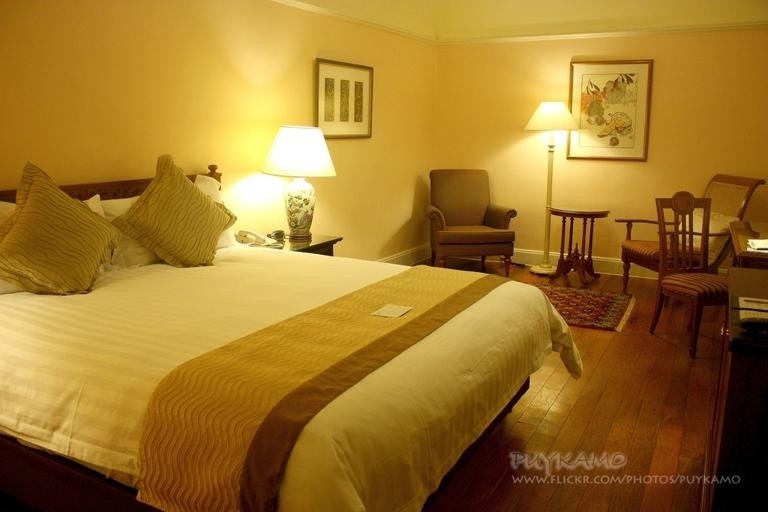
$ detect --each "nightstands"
[272,233,347,256]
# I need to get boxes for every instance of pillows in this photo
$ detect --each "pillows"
[2,153,242,296]
[672,208,740,265]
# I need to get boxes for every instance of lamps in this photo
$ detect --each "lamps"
[521,101,573,272]
[257,124,335,243]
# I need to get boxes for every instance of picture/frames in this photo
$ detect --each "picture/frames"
[312,56,375,139]
[565,59,656,162]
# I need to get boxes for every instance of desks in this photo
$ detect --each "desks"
[702,268,767,512]
[729,221,767,268]
[548,205,607,287]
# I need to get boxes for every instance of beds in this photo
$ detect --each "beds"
[1,163,586,512]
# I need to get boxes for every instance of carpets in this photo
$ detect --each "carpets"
[522,284,637,335]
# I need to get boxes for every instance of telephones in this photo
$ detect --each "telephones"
[234,229,284,250]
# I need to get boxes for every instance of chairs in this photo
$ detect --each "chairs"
[427,167,514,278]
[616,173,765,295]
[653,191,728,356]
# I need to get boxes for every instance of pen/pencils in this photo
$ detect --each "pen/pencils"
[757,248,768,250]
[734,306,764,312]
[744,299,768,304]
